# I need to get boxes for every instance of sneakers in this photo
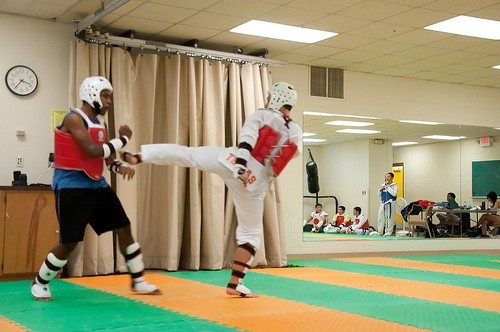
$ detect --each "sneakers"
[30,281,52,300]
[131,280,160,294]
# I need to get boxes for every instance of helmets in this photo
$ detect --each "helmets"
[80,76,113,110]
[267,82,298,110]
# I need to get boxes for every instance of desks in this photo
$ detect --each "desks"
[425,209,496,238]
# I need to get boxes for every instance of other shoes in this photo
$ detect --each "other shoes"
[476,235,486,239]
[468,226,477,232]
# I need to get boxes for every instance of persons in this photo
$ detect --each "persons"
[377,172,398,236]
[31,76,161,301]
[303,204,370,235]
[423,193,460,238]
[469,191,500,238]
[119,82,302,297]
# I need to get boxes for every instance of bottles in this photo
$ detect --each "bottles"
[463,201,467,210]
[481,201,485,210]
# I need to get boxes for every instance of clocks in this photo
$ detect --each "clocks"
[5,65,38,96]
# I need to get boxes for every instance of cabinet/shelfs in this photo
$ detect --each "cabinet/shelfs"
[0,186,62,280]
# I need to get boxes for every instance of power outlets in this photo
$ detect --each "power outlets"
[48,161,55,168]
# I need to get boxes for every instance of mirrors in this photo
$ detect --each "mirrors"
[302,112,500,241]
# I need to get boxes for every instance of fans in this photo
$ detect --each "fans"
[395,197,412,235]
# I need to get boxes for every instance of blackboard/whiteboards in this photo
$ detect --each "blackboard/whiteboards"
[470,159,499,199]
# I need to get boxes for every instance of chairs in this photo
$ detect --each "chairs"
[406,204,459,238]
